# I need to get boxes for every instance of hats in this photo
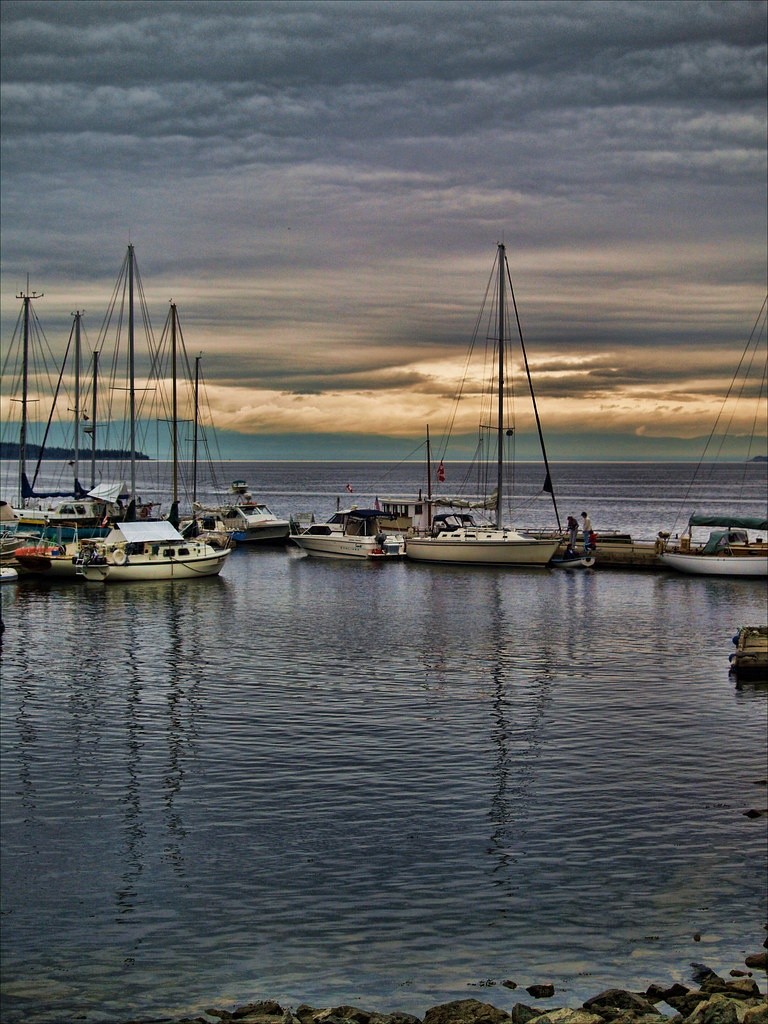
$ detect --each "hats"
[193,500,201,508]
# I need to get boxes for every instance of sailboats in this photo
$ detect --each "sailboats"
[0,233,768,588]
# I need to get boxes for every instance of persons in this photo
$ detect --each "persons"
[565,516,579,548]
[563,543,577,560]
[580,512,598,551]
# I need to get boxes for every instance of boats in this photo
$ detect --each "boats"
[726,624,767,681]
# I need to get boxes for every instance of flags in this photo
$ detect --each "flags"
[436,459,445,483]
[346,484,352,493]
